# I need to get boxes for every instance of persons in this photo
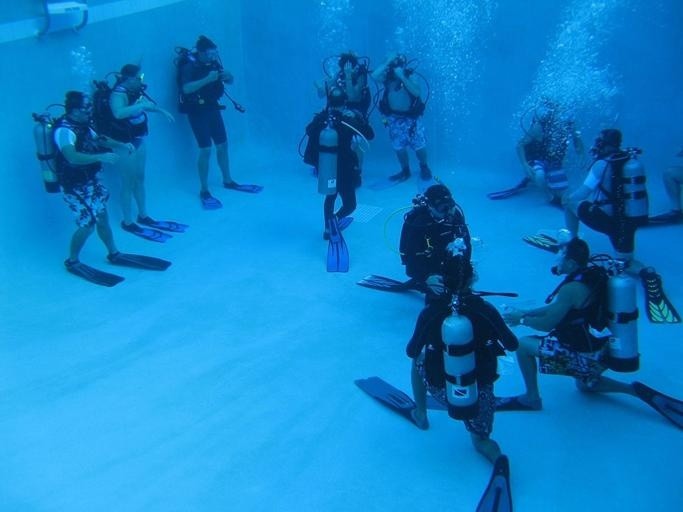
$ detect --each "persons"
[372,53,432,182]
[515,108,584,203]
[51,91,118,263]
[400,184,471,304]
[109,64,146,226]
[503,236,639,410]
[411,257,519,464]
[180,36,233,194]
[303,53,374,240]
[564,128,646,276]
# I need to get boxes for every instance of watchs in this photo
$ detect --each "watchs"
[519,315,526,326]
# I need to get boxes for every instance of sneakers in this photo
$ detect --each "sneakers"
[639,266,663,294]
[632,380,660,406]
[550,195,562,205]
[521,178,531,186]
[403,403,429,429]
[64,258,80,273]
[223,181,236,188]
[389,168,412,182]
[121,215,154,232]
[323,213,340,242]
[200,190,210,199]
[508,396,542,410]
[106,252,119,263]
[420,164,432,180]
[492,456,509,486]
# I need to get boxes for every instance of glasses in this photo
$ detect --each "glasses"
[594,136,611,147]
[432,200,455,213]
[78,103,93,113]
[133,73,145,83]
[203,52,218,59]
